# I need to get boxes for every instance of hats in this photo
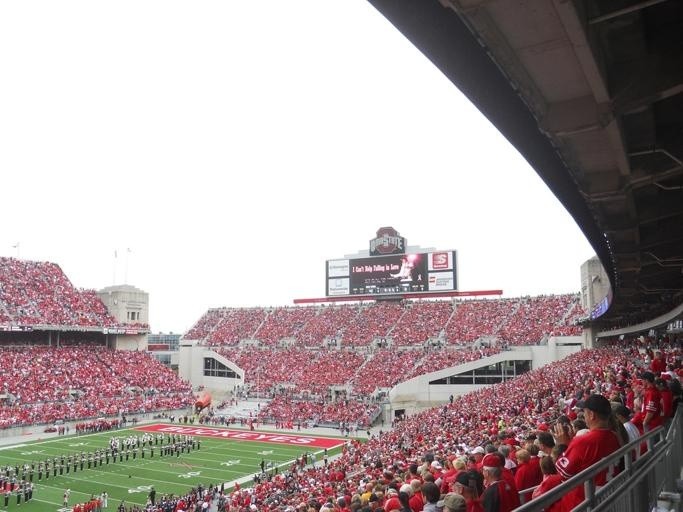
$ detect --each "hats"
[477,454,503,470]
[576,395,612,414]
[437,492,467,512]
[471,446,485,454]
[638,372,654,381]
[431,461,443,469]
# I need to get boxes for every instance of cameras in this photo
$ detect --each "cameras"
[554,422,573,435]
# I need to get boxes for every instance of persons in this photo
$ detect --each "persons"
[0,432,201,512]
[179,291,585,438]
[200,338,683,512]
[1,339,205,436]
[389,256,414,279]
[0,255,150,329]
